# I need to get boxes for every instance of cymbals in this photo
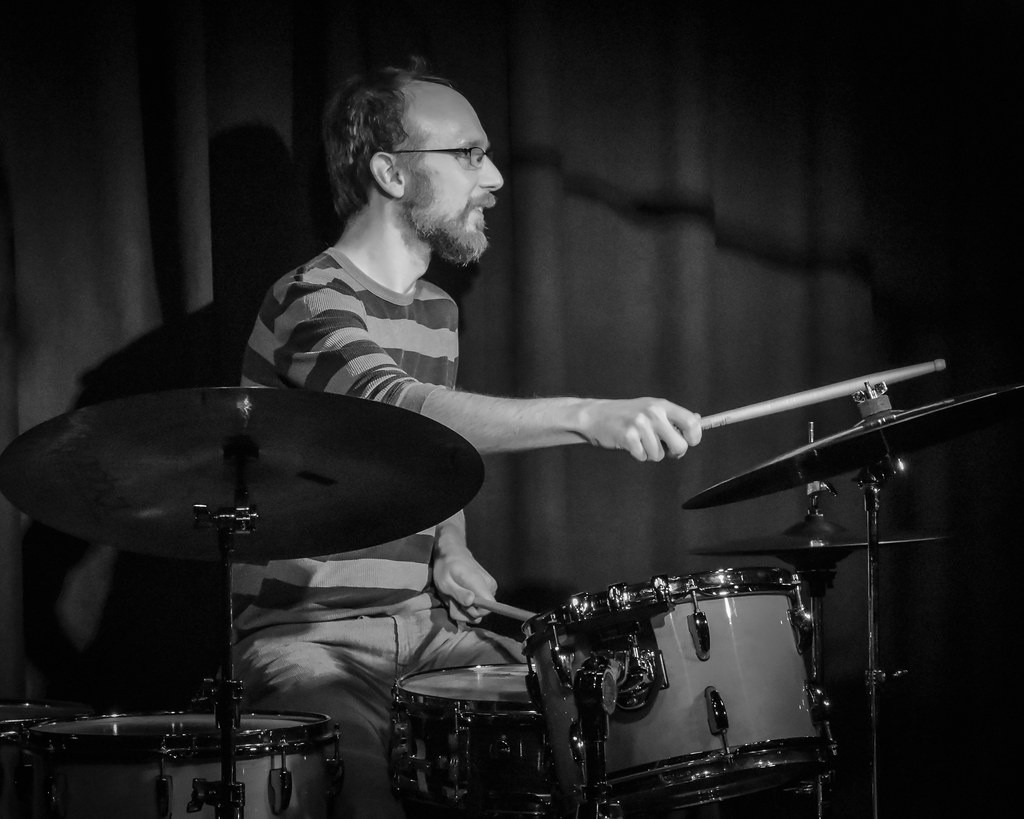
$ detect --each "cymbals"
[679,386,1024,512]
[838,421,841,426]
[690,519,945,565]
[1,387,484,564]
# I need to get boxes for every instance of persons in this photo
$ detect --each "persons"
[226,60,706,819]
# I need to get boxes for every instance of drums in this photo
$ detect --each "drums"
[30,707,341,819]
[0,700,79,819]
[522,564,839,819]
[391,663,550,817]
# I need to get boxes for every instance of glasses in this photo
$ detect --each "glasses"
[388,147,487,168]
